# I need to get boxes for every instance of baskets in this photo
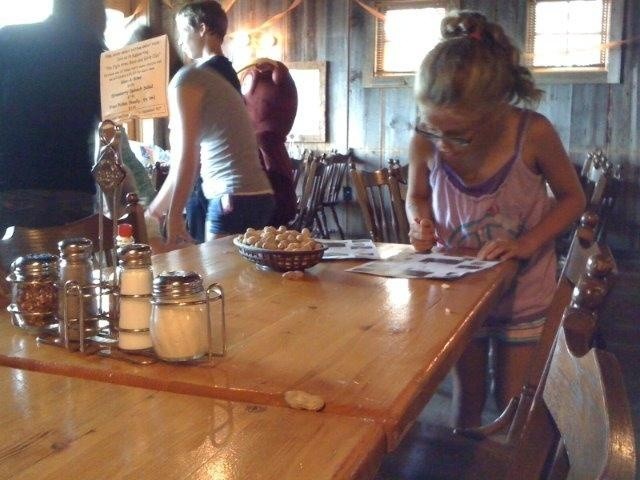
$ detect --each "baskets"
[233,237,328,273]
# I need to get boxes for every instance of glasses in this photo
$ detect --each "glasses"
[415,118,480,145]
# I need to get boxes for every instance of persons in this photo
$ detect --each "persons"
[0,0,110,241]
[174,0,243,245]
[406,10,588,443]
[126,27,276,245]
[273,252,276,258]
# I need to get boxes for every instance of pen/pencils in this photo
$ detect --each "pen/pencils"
[413,216,448,247]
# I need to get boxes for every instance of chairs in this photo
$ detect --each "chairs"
[384,148,637,479]
[142,146,383,244]
[0,188,154,303]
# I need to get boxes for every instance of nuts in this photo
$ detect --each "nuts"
[236,226,322,251]
[284,390,324,411]
[282,270,304,279]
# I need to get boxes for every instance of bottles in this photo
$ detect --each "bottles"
[58,237,101,342]
[150,268,209,364]
[117,244,157,353]
[9,254,63,333]
[109,223,140,337]
